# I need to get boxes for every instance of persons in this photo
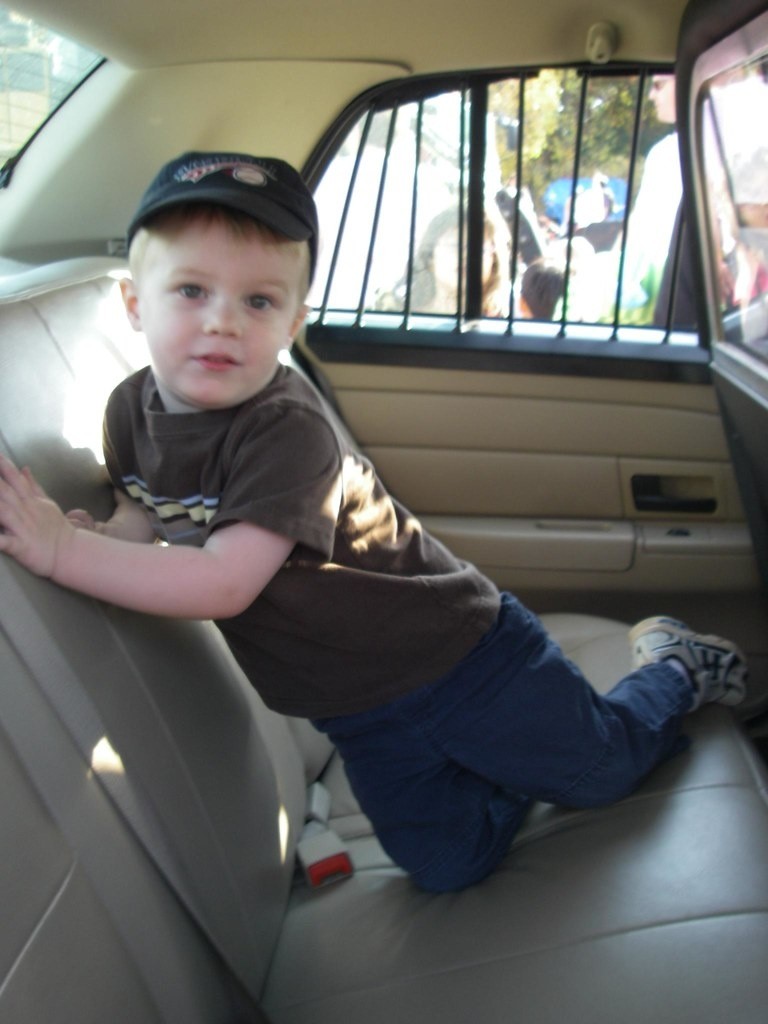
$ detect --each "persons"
[565,74,683,324]
[505,174,517,191]
[0,151,749,896]
[521,258,565,320]
[383,203,504,318]
[590,171,613,221]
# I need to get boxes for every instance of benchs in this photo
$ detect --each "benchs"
[0,255,768,1024]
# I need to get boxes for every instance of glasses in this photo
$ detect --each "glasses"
[653,79,669,90]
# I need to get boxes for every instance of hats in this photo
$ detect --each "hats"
[128,150,316,284]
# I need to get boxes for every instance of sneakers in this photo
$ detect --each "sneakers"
[628,617,752,712]
